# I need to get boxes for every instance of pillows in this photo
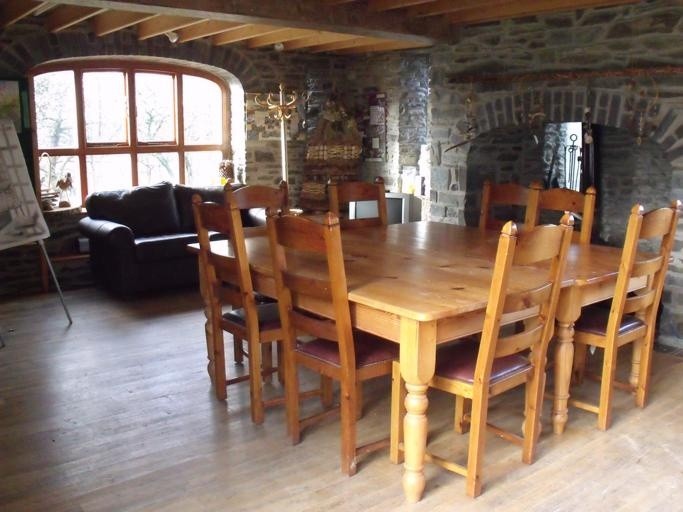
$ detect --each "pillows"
[174,183,256,227]
[86,182,174,236]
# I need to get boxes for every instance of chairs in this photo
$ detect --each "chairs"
[38,209,93,294]
[224,179,293,382]
[191,193,333,426]
[266,206,400,476]
[325,177,388,227]
[392,210,573,496]
[459,179,595,353]
[524,197,680,430]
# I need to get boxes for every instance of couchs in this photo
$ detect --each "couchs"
[76,181,256,297]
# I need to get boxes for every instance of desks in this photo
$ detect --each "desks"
[186,219,649,503]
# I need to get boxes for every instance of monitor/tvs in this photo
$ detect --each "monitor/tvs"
[349,192,410,224]
